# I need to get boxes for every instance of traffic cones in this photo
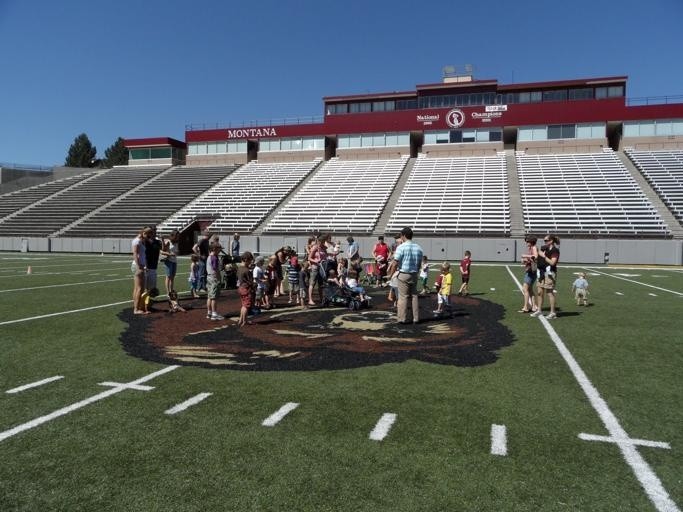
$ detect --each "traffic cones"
[25,266,32,275]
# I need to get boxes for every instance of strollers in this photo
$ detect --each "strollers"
[319,259,363,310]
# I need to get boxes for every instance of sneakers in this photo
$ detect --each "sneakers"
[238,323,241,326]
[530,311,542,317]
[206,314,211,318]
[200,289,205,293]
[365,296,371,300]
[288,300,292,303]
[274,295,279,297]
[145,311,150,314]
[464,293,470,296]
[419,292,425,295]
[280,293,287,296]
[261,304,266,308]
[433,310,442,313]
[267,304,270,307]
[396,322,405,326]
[134,310,142,315]
[459,292,463,296]
[361,299,365,302]
[211,315,224,321]
[428,289,432,293]
[413,322,419,326]
[242,323,248,326]
[308,302,316,305]
[194,295,199,297]
[297,300,299,303]
[546,313,557,320]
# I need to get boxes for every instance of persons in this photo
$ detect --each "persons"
[530,232,561,320]
[144,225,166,292]
[130,227,152,316]
[517,235,538,313]
[458,250,473,296]
[187,228,422,327]
[419,255,430,297]
[160,228,182,294]
[137,288,159,315]
[167,290,186,315]
[433,261,452,314]
[537,245,553,279]
[573,272,589,306]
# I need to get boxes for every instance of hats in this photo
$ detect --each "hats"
[376,255,384,262]
[212,242,225,249]
[254,256,265,264]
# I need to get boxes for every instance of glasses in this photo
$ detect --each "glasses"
[544,240,551,242]
[525,241,530,243]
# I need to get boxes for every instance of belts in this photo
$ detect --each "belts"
[399,272,417,275]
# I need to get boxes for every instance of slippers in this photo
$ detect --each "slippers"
[517,310,529,313]
[528,308,537,312]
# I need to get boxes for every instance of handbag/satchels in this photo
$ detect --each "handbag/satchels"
[160,254,167,262]
